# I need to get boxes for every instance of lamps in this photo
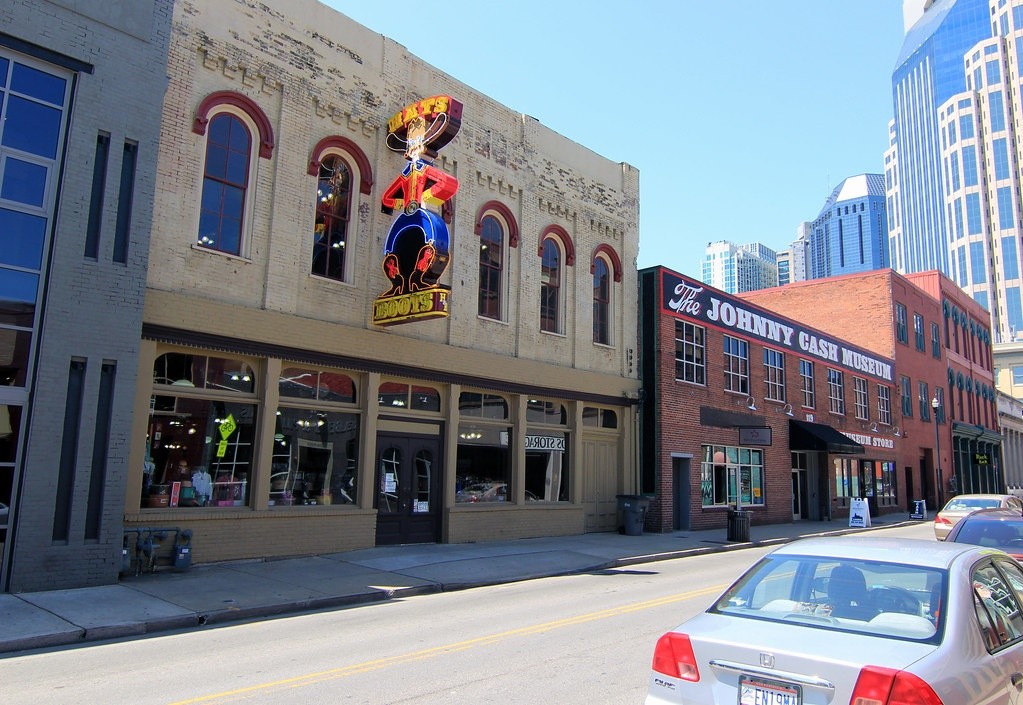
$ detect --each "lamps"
[863,422,878,432]
[886,426,900,436]
[776,404,794,417]
[744,397,757,411]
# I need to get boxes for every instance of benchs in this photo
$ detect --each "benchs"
[760,599,937,639]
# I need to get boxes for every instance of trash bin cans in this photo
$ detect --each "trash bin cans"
[615,495,655,536]
[727,510,753,542]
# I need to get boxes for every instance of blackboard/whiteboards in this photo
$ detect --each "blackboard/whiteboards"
[909,500,928,519]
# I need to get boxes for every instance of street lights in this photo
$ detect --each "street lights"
[932,397,942,514]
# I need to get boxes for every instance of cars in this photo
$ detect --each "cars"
[946,508,1022,565]
[269,471,309,495]
[645,534,1023,705]
[933,494,1022,543]
[456,483,540,503]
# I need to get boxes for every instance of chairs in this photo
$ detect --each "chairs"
[824,566,873,621]
